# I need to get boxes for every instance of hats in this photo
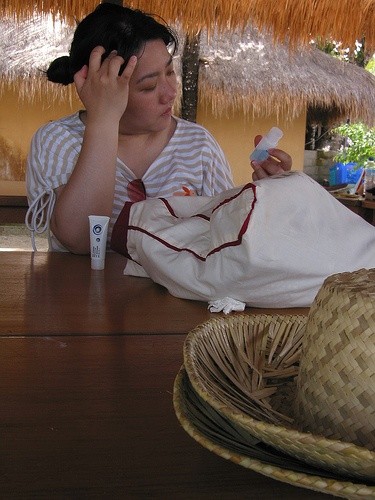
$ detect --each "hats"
[172,268,374,500]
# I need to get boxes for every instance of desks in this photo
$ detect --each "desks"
[0,251,339,500]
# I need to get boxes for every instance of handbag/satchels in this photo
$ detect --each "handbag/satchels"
[111,171,375,309]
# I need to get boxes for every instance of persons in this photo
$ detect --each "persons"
[24,3,294,255]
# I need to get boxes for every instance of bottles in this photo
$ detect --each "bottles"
[249,127,283,162]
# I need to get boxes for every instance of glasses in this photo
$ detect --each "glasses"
[127,178,146,202]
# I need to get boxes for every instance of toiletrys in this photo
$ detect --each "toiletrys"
[87,215,110,272]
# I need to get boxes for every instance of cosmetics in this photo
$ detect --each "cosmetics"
[88,215,110,270]
[249,127,283,164]
